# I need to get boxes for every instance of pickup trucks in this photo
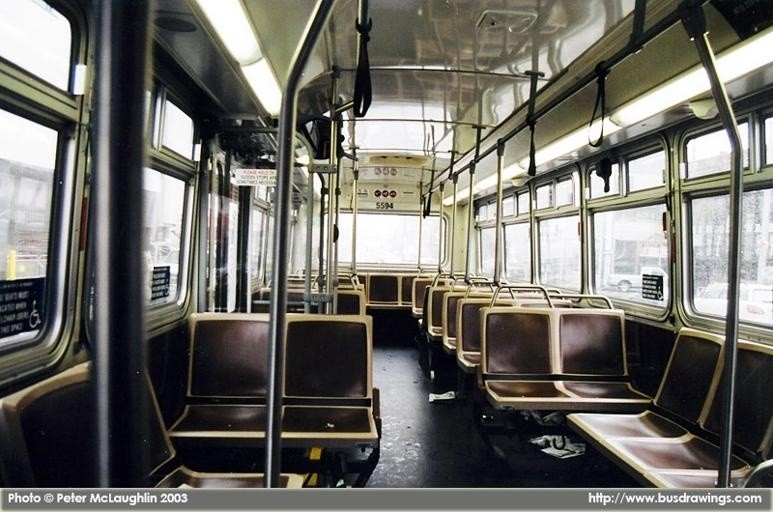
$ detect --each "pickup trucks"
[605,266,668,293]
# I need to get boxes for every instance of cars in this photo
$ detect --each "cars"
[695,282,773,323]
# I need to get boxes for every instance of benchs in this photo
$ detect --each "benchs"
[296,272,476,309]
[0,272,381,488]
[412,272,726,487]
